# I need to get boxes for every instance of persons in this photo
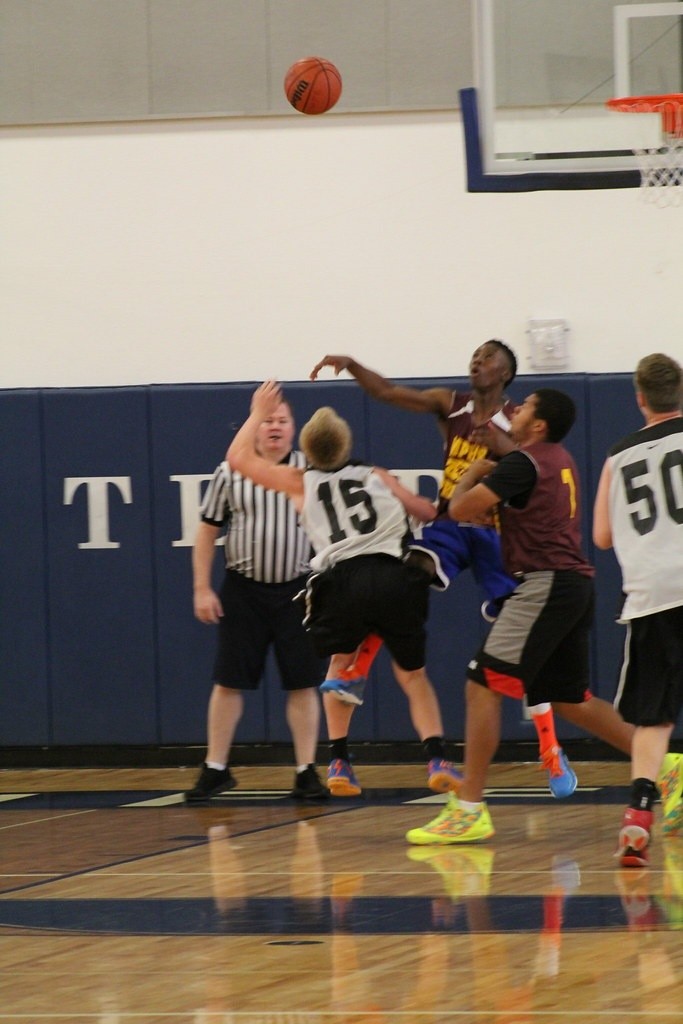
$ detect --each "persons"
[226,380,463,796]
[403,389,683,843]
[591,353,683,866]
[181,397,334,800]
[191,803,683,1024]
[310,339,578,799]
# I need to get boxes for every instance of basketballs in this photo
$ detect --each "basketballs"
[283,56,344,116]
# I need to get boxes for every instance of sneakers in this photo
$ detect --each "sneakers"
[656,752,683,837]
[405,845,495,897]
[539,747,578,799]
[184,762,238,800]
[426,760,464,792]
[405,790,496,845]
[326,759,362,797]
[290,763,331,801]
[619,807,654,867]
[319,679,368,705]
